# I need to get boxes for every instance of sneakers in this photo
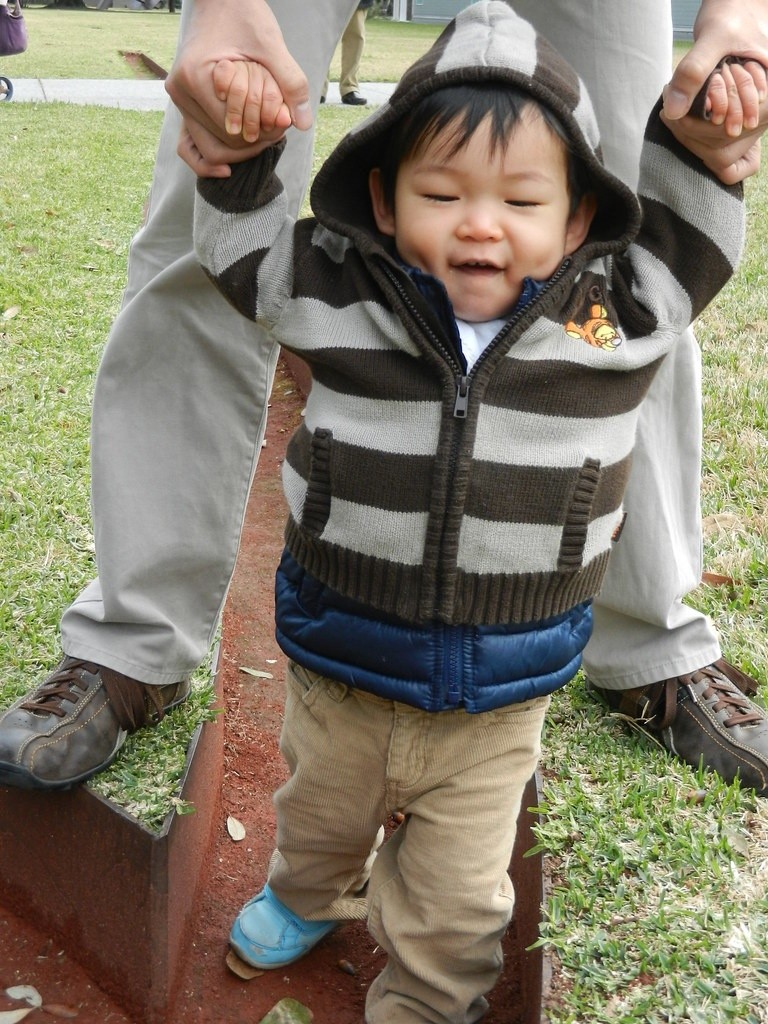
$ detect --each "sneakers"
[585,659,766,797]
[0,654,194,785]
[228,878,368,970]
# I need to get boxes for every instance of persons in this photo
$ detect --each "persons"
[1,0,767,800]
[319,0,371,104]
[191,57,767,1023]
